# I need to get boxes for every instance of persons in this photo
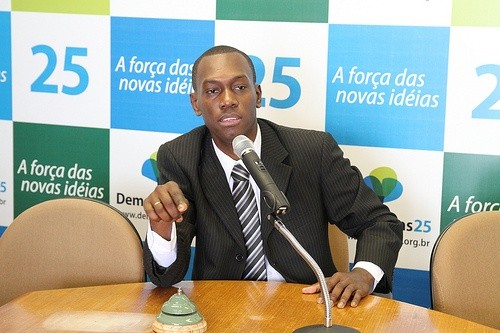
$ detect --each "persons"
[143,46,403,309]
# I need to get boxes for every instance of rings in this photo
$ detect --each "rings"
[154,202,160,205]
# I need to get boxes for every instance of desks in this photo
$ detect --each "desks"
[0,273,500,333]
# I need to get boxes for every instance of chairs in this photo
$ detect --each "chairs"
[0,197,148,306]
[430,209,500,330]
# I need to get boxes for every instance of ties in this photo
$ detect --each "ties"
[231,163,267,282]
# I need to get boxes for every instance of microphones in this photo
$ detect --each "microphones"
[232,135,289,215]
